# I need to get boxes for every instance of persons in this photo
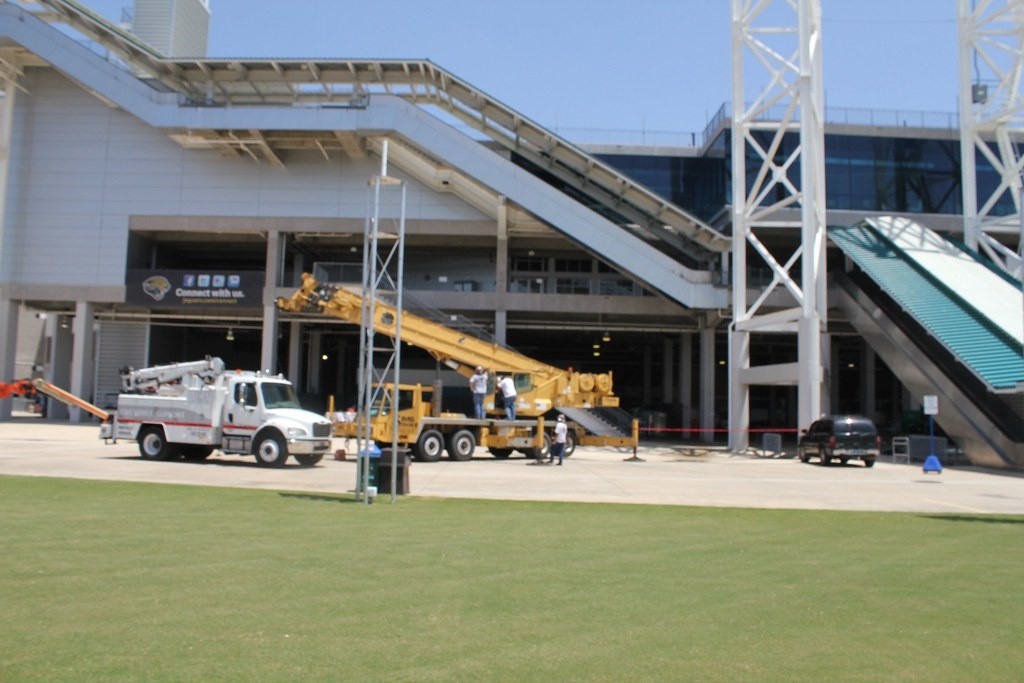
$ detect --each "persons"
[497,376,517,420]
[469,366,488,419]
[546,413,568,465]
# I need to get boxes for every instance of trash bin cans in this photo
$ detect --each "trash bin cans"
[360,442,411,495]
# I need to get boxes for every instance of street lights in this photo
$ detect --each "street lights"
[366,176,408,507]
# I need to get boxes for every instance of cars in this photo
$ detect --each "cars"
[797,414,882,467]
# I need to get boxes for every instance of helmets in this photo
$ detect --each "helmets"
[476,366,482,370]
[497,377,501,383]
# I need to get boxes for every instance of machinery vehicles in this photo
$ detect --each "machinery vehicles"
[274,270,648,465]
[0,353,336,470]
